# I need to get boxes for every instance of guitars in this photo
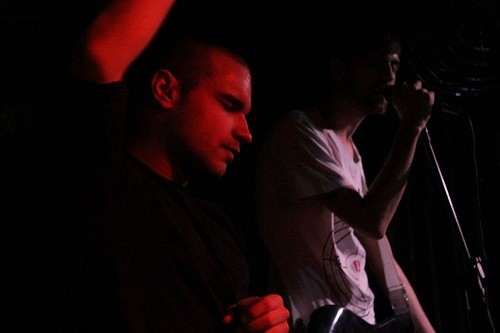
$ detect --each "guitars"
[305,302,414,333]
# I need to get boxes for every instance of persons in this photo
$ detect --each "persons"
[83,1,296,333]
[255,23,438,333]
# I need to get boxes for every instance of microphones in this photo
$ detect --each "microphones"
[235,296,263,327]
[392,84,469,118]
